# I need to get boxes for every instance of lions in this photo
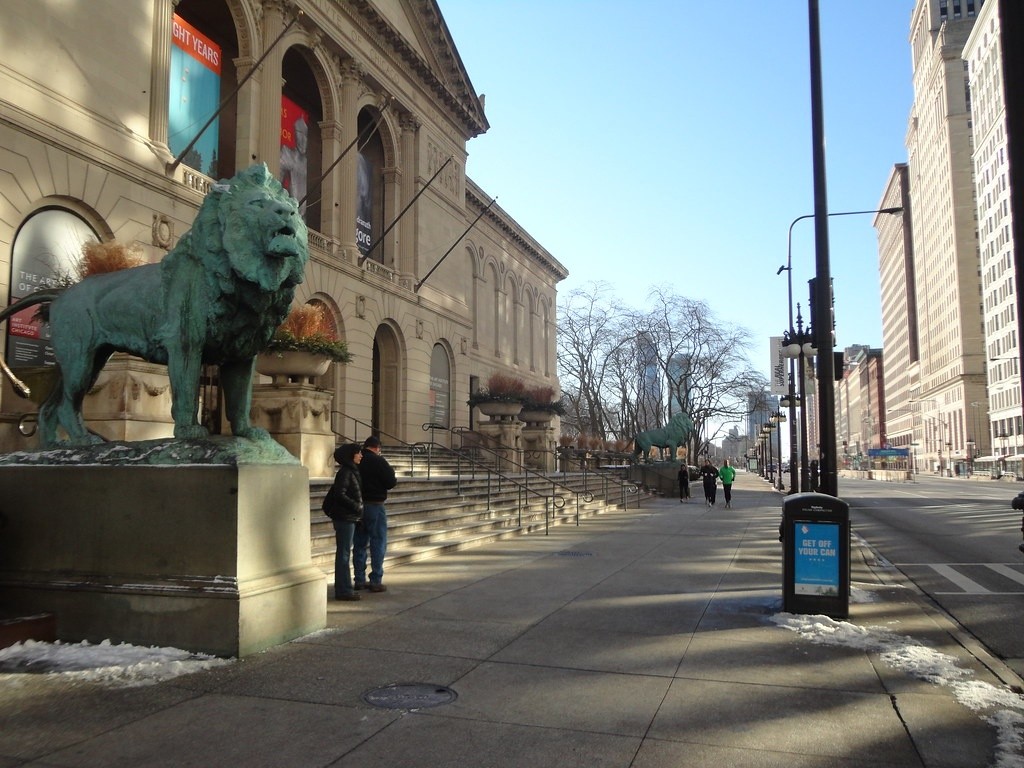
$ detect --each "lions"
[621,412,693,464]
[0,162,308,447]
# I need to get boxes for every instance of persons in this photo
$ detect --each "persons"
[699,459,719,507]
[352,436,397,593]
[330,444,365,601]
[719,460,736,509]
[677,464,689,503]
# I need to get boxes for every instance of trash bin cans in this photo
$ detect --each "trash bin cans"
[778,492,852,619]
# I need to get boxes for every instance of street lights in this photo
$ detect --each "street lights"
[998,429,1009,476]
[888,400,947,477]
[777,208,905,494]
[745,406,787,490]
[966,438,976,474]
[946,441,952,477]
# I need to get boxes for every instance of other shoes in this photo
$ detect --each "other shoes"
[354,581,370,591]
[349,591,361,600]
[369,584,387,592]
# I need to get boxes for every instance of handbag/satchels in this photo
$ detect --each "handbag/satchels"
[322,484,349,523]
